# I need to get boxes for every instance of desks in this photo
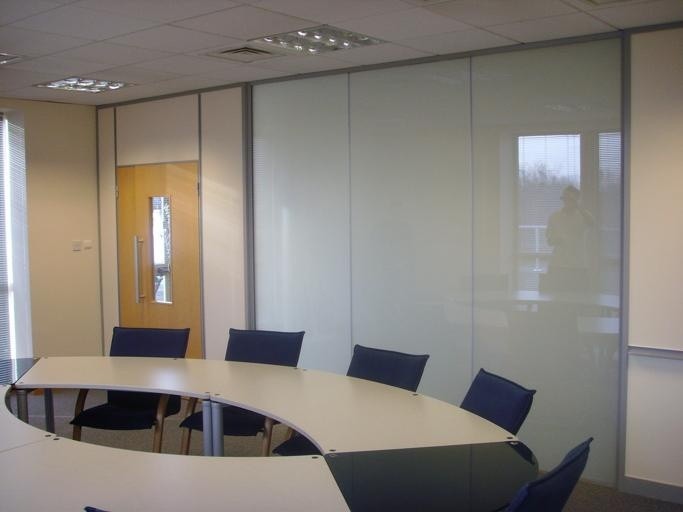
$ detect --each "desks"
[0,356,539,512]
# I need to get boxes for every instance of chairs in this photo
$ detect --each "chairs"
[69,326,189,453]
[459,368,536,436]
[271,343,429,457]
[504,437,594,512]
[179,329,305,457]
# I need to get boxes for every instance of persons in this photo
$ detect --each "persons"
[545,185,599,290]
